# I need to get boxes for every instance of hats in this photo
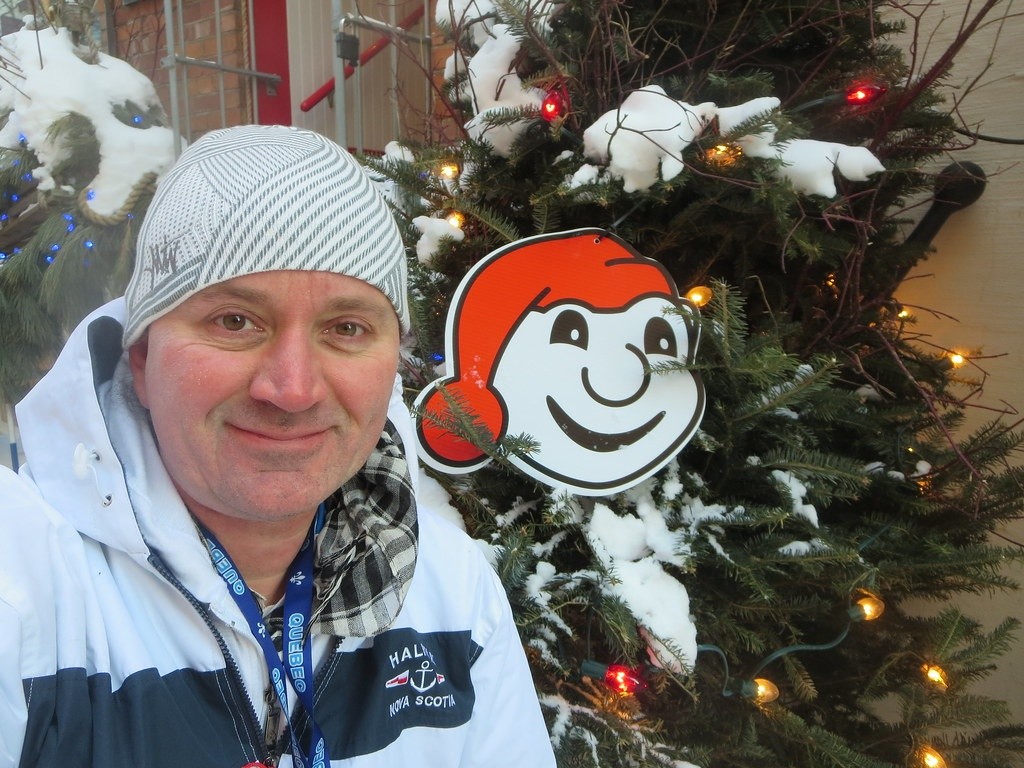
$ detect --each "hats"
[121,125,411,350]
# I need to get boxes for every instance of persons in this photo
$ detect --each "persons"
[0,123,558,768]
